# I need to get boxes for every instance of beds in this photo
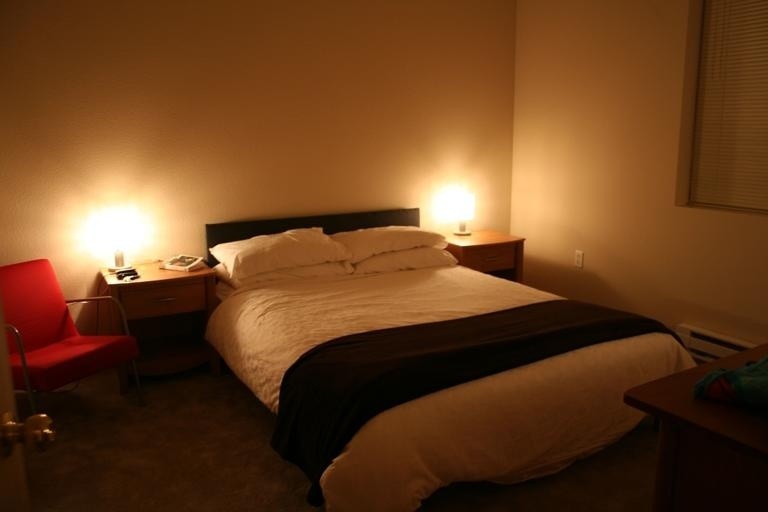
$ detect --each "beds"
[206,208,697,512]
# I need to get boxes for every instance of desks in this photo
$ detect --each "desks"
[623,344,768,511]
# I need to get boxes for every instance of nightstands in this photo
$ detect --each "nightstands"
[101,257,219,391]
[445,230,526,283]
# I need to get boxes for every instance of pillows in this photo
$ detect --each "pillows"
[210,227,350,279]
[327,225,446,264]
[213,262,354,290]
[353,247,458,274]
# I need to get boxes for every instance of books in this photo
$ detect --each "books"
[161,255,206,272]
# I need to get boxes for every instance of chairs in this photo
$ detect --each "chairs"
[0,259,145,415]
